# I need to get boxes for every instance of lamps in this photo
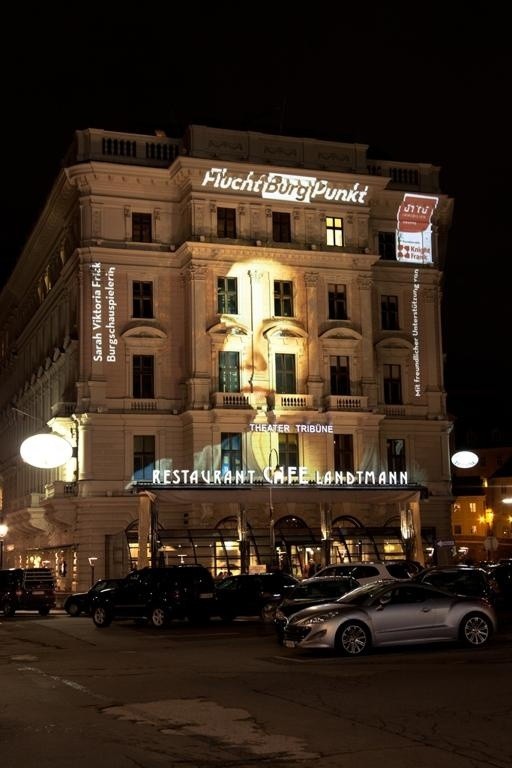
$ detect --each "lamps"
[18,421,74,470]
[448,446,480,471]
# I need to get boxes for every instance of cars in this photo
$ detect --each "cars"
[0,568,56,617]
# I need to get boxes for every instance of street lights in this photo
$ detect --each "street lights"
[88,558,97,586]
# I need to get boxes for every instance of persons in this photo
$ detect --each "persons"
[448,546,457,560]
[302,559,314,579]
